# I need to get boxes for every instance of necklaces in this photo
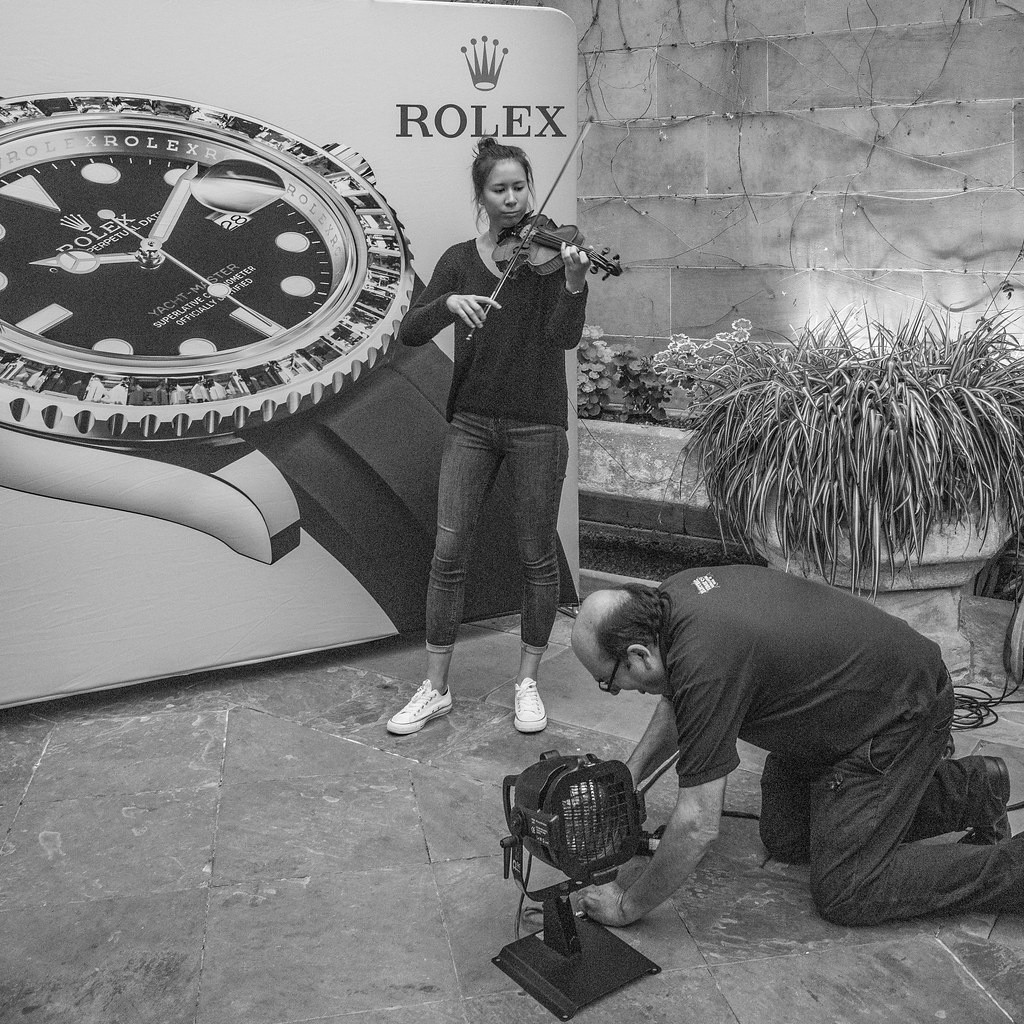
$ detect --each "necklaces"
[488,228,495,249]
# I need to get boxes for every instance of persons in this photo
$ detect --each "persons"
[387,137,591,734]
[571,565,1024,927]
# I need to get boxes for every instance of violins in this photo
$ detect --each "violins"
[490,211,624,283]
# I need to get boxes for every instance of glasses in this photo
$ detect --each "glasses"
[597,655,623,695]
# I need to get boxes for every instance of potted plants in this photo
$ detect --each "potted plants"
[670,301,1024,593]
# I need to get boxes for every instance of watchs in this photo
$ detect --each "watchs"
[572,290,582,295]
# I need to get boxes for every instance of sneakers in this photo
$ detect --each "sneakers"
[387,678,452,736]
[513,677,547,733]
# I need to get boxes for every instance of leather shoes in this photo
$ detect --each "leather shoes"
[957,755,1011,844]
